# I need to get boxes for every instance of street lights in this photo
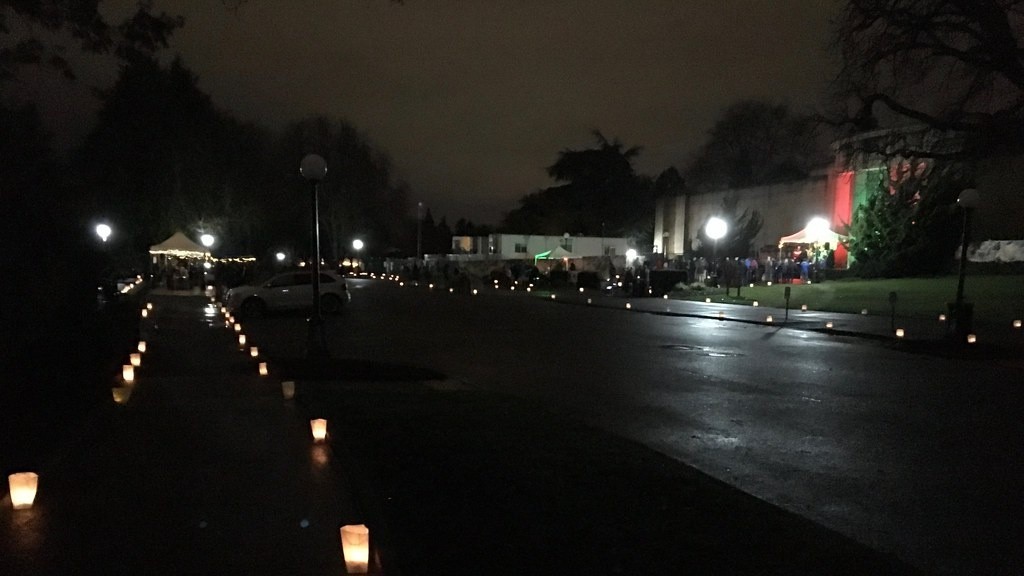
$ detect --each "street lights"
[415,201,422,258]
[564,232,570,251]
[701,213,732,276]
[945,190,978,314]
[301,155,327,364]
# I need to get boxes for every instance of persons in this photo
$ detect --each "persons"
[570,263,577,279]
[403,264,537,289]
[609,263,617,294]
[624,243,835,296]
[118,259,245,295]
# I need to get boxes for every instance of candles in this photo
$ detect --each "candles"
[122,365,134,382]
[310,418,327,440]
[259,362,268,375]
[282,382,294,398]
[9,472,39,508]
[130,354,141,367]
[339,524,369,576]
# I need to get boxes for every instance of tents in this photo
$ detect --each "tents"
[780,228,856,262]
[148,232,212,285]
[535,247,583,272]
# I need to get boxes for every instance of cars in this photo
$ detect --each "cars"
[225,269,351,319]
